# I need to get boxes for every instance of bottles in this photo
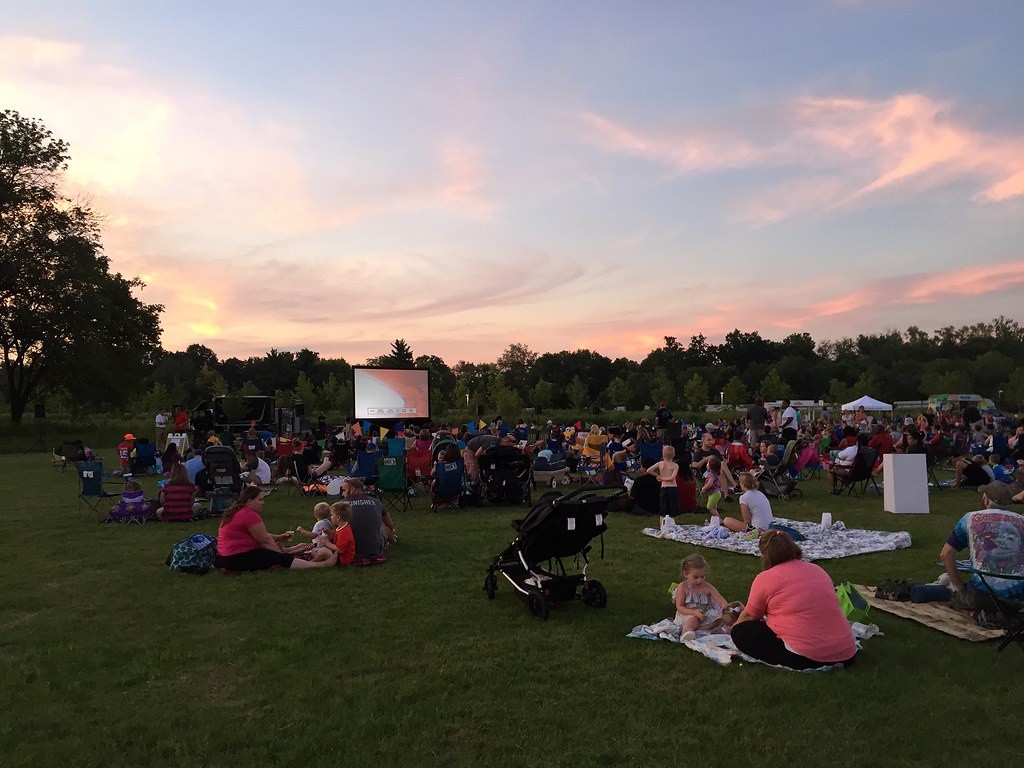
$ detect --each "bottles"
[850,620,871,634]
[661,515,675,531]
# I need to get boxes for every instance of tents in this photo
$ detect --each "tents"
[840,395,895,420]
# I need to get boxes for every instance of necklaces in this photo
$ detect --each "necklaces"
[336,522,349,532]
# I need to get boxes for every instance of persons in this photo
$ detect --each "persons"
[731,531,858,668]
[646,445,680,529]
[724,474,773,531]
[309,501,356,567]
[55,394,1024,510]
[700,457,723,525]
[276,501,334,554]
[941,482,1024,623]
[341,478,397,558]
[676,555,728,642]
[218,486,338,570]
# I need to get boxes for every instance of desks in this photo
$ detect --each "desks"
[883,454,930,513]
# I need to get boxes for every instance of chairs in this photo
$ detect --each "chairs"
[926,460,943,491]
[832,442,882,499]
[118,490,148,526]
[935,560,1024,653]
[127,442,471,512]
[1000,449,1021,481]
[73,461,123,524]
[573,428,766,500]
[56,440,90,472]
[796,438,831,480]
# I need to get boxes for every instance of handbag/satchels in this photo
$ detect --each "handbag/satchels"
[875,577,912,601]
[768,523,806,542]
[835,580,870,623]
[169,534,218,570]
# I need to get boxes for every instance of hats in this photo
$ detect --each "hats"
[124,433,136,440]
[978,479,1013,505]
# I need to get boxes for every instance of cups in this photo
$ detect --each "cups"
[821,513,831,528]
[168,433,186,437]
[287,531,294,541]
[711,516,719,526]
[703,470,709,478]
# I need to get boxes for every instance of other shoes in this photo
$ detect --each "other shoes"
[680,631,696,642]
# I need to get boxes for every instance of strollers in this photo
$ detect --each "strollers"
[748,440,803,503]
[482,483,628,621]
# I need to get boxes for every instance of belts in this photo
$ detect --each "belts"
[156,427,164,429]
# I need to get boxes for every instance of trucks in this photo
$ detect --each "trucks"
[187,394,276,436]
